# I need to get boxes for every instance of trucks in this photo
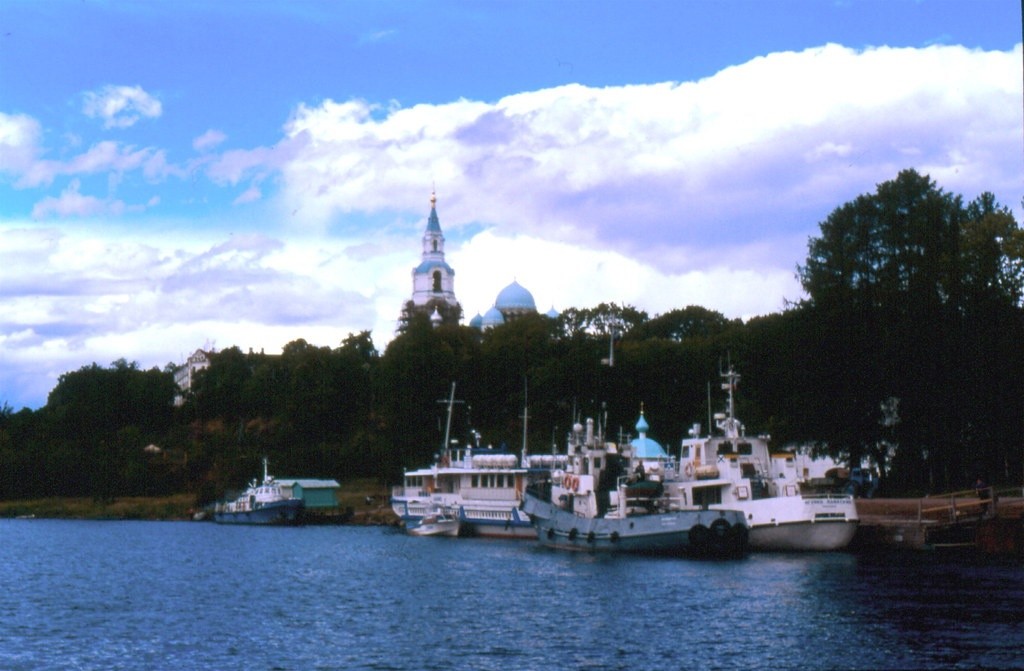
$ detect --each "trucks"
[810,467,871,494]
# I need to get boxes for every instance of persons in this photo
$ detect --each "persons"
[976,479,988,510]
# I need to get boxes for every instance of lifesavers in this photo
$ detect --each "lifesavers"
[572,478,578,491]
[565,476,571,490]
[688,518,748,548]
[685,462,692,477]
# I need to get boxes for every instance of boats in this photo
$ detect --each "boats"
[408,495,467,539]
[642,350,859,554]
[390,380,538,539]
[518,399,749,561]
[191,511,209,521]
[203,457,306,525]
